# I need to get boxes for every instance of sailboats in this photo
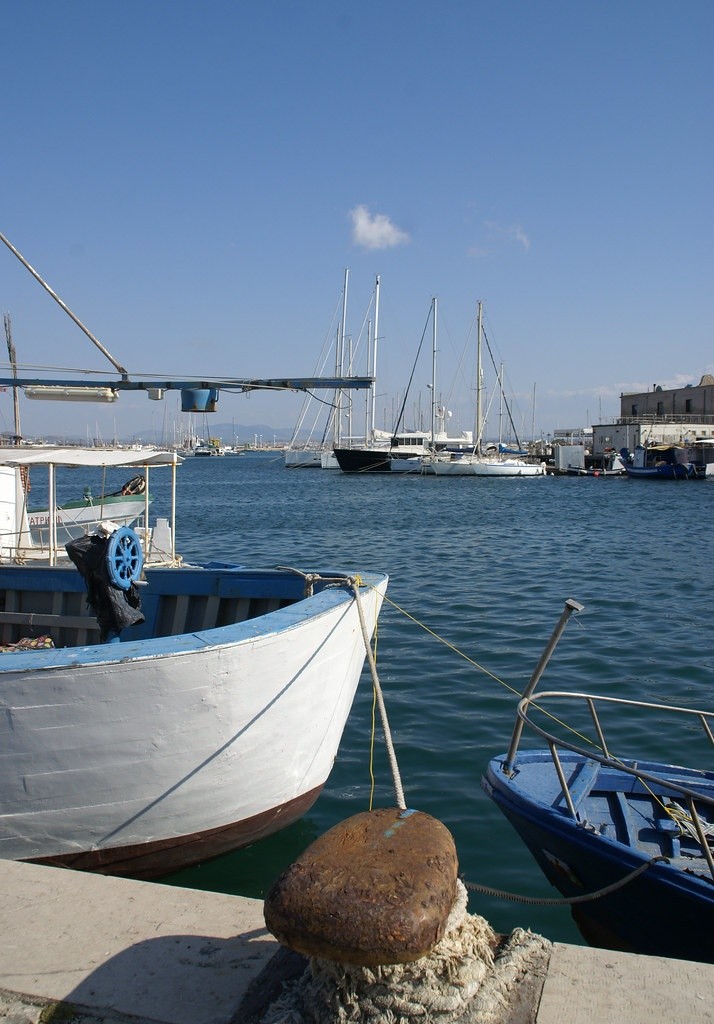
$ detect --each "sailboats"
[282,265,546,474]
[83,398,246,457]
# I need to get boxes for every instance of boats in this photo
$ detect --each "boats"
[0,230,390,877]
[477,596,713,963]
[567,459,697,480]
